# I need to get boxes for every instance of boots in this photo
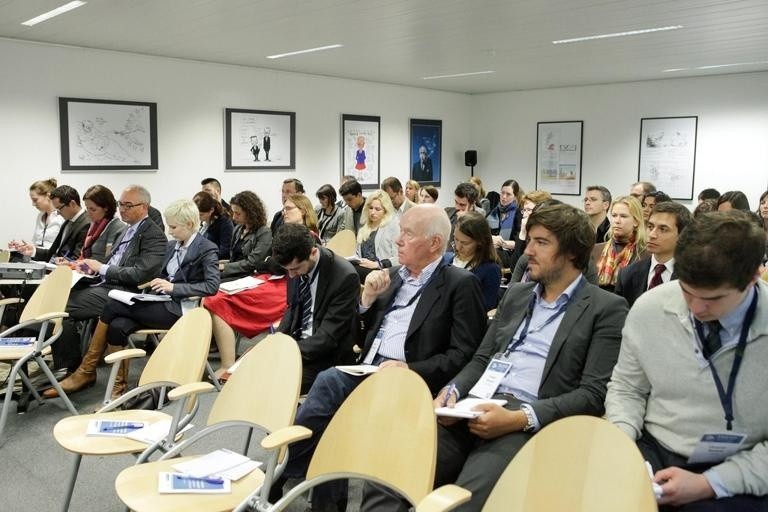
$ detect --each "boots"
[107,345,129,399]
[57,320,110,392]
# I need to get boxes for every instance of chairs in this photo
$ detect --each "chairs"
[262,363,472,512]
[53,307,216,510]
[115,331,312,512]
[1,262,79,451]
[1,227,367,400]
[482,414,660,512]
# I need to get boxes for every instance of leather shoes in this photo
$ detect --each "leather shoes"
[44,387,59,398]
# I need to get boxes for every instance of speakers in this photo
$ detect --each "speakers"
[465,150,477,166]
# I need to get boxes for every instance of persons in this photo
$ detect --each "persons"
[413,145,432,181]
[264,128,271,161]
[355,137,366,181]
[0,175,768,510]
[250,137,261,162]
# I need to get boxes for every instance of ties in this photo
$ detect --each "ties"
[702,320,722,359]
[298,278,312,329]
[648,263,667,290]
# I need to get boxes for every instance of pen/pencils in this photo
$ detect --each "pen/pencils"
[376,257,383,271]
[142,289,145,297]
[270,322,275,334]
[177,477,224,484]
[5,342,35,344]
[104,424,144,430]
[437,382,455,420]
[62,250,70,262]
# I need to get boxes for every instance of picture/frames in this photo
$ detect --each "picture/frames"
[637,116,699,200]
[59,97,158,171]
[339,114,381,189]
[534,120,584,196]
[410,117,443,189]
[223,108,296,172]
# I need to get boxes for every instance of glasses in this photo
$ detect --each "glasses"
[115,201,143,210]
